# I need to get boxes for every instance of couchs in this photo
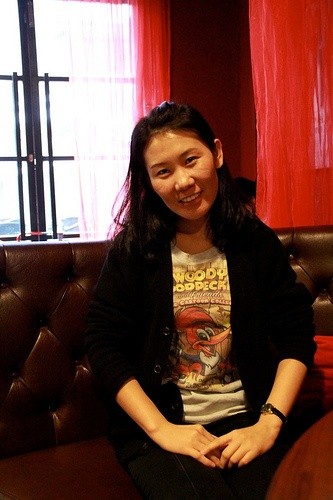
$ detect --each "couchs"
[0,224,332,500]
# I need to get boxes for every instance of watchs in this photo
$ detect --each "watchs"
[260,402,288,424]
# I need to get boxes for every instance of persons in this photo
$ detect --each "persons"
[88,104,324,500]
